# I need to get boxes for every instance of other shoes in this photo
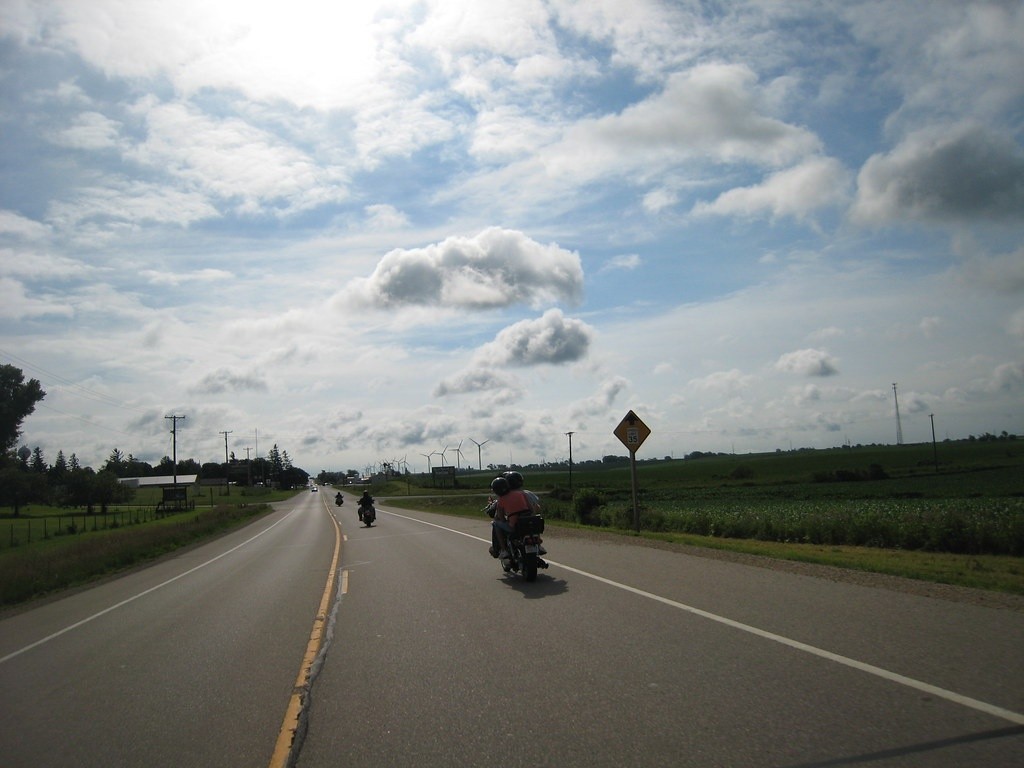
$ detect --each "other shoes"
[539,547,546,555]
[489,547,499,558]
[499,552,509,559]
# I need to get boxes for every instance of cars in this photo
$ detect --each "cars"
[312,487,318,492]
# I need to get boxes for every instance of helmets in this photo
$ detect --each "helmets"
[503,471,524,489]
[490,477,510,496]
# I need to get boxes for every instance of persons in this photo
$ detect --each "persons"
[486,471,547,558]
[357,490,376,521]
[334,492,344,504]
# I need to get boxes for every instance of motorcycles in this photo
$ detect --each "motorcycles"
[356,500,376,527]
[480,499,549,582]
[335,496,343,506]
